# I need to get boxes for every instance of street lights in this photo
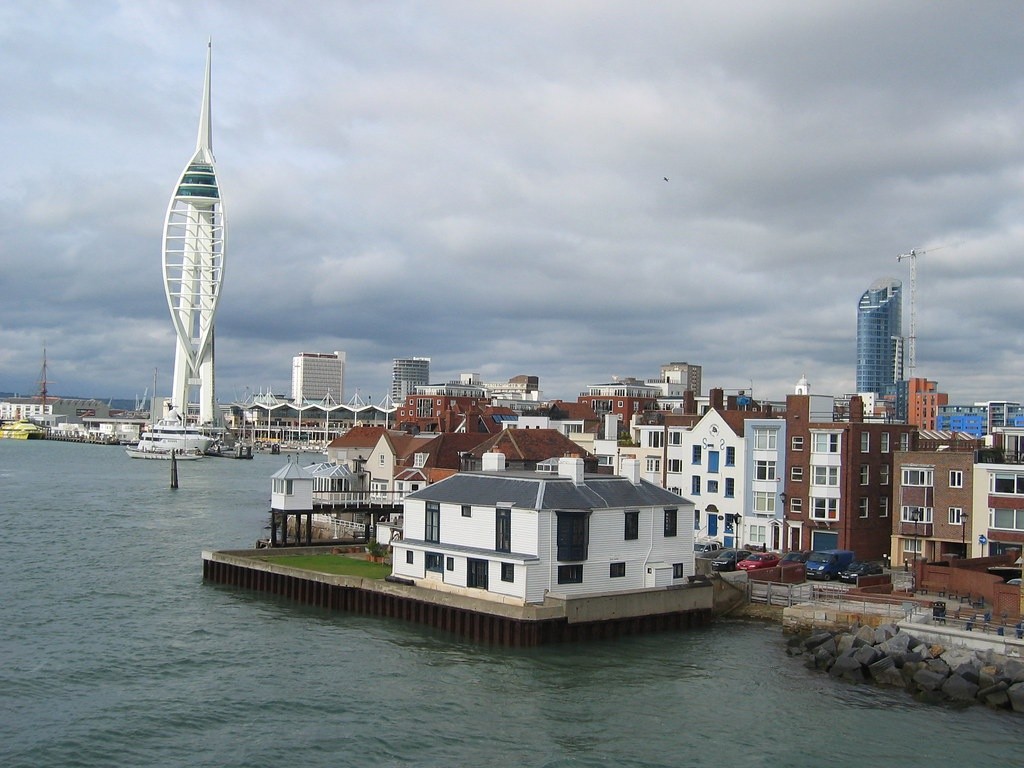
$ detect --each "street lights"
[734,512,742,549]
[911,509,922,571]
[960,512,969,559]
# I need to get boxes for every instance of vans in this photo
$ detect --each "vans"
[804,549,857,581]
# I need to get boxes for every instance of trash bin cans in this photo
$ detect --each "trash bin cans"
[933,601,946,621]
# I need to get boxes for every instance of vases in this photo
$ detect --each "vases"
[354,547,361,553]
[347,548,352,553]
[332,548,339,554]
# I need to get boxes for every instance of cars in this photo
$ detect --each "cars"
[736,551,785,571]
[775,550,812,580]
[838,559,883,585]
[694,539,757,572]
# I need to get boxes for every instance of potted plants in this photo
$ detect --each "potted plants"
[364,537,390,564]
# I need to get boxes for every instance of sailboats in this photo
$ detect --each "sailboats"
[0,415,51,441]
[123,367,329,461]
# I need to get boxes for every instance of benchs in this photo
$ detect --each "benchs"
[353,531,374,541]
[934,609,976,630]
[999,615,1024,639]
[966,615,1008,636]
[919,581,946,597]
[949,587,972,603]
[969,595,984,609]
[955,606,990,622]
